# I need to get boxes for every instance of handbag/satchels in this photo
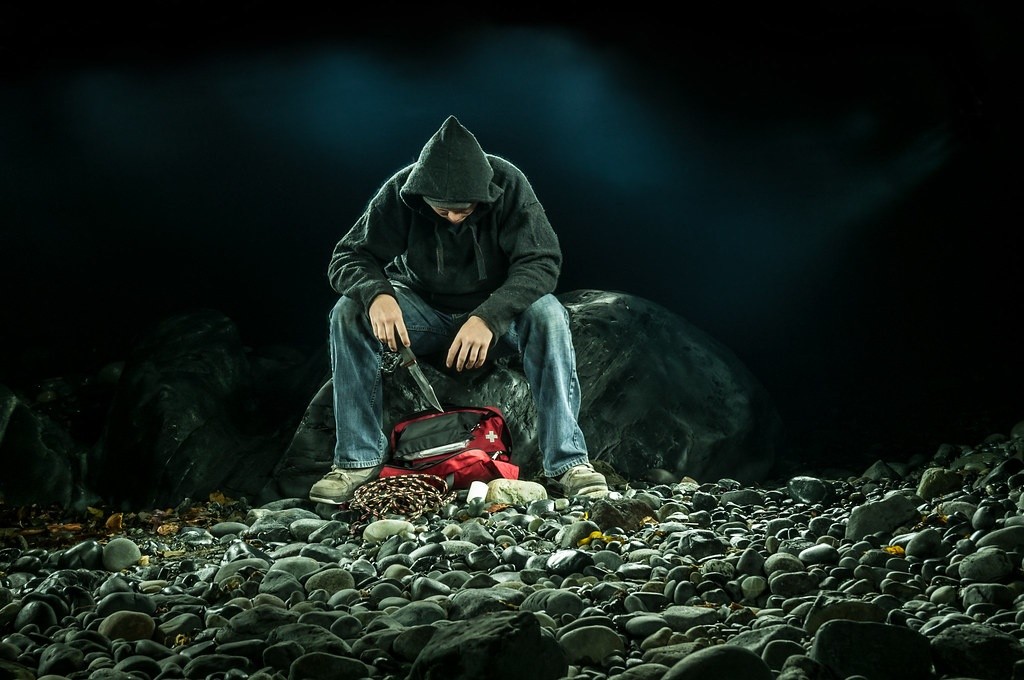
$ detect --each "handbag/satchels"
[379,406,519,484]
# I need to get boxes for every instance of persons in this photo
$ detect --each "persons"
[307,115,608,513]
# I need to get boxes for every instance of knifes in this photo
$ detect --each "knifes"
[394,325,444,412]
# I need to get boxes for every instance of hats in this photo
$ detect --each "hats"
[424,196,471,208]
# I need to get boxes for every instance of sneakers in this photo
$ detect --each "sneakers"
[310,465,383,504]
[558,465,608,497]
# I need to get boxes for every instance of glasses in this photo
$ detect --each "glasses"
[433,202,479,216]
